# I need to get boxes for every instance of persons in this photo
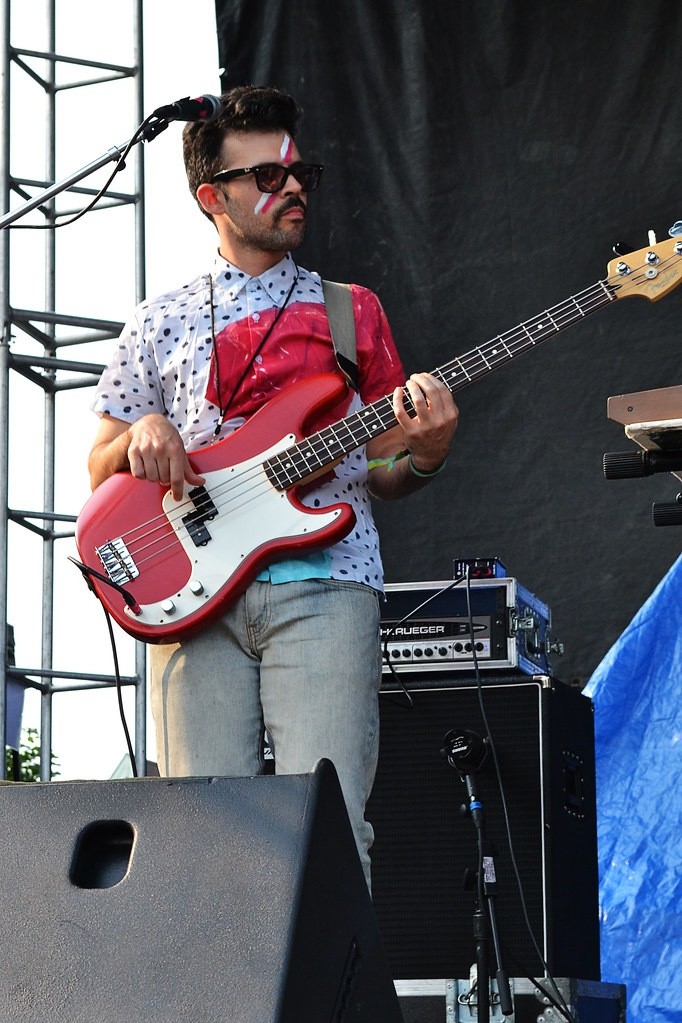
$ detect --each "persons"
[86,84,459,899]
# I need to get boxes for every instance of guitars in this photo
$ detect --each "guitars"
[64,219,682,647]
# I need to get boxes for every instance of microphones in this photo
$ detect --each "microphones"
[152,95,223,123]
[443,726,494,771]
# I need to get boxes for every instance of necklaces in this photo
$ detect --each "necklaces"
[208,273,297,438]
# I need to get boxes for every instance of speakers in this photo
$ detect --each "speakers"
[0,672,595,1023]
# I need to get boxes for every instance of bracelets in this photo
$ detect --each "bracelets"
[409,456,447,478]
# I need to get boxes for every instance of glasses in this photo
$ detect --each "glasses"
[210,162,325,193]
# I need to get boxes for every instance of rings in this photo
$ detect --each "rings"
[160,482,170,486]
